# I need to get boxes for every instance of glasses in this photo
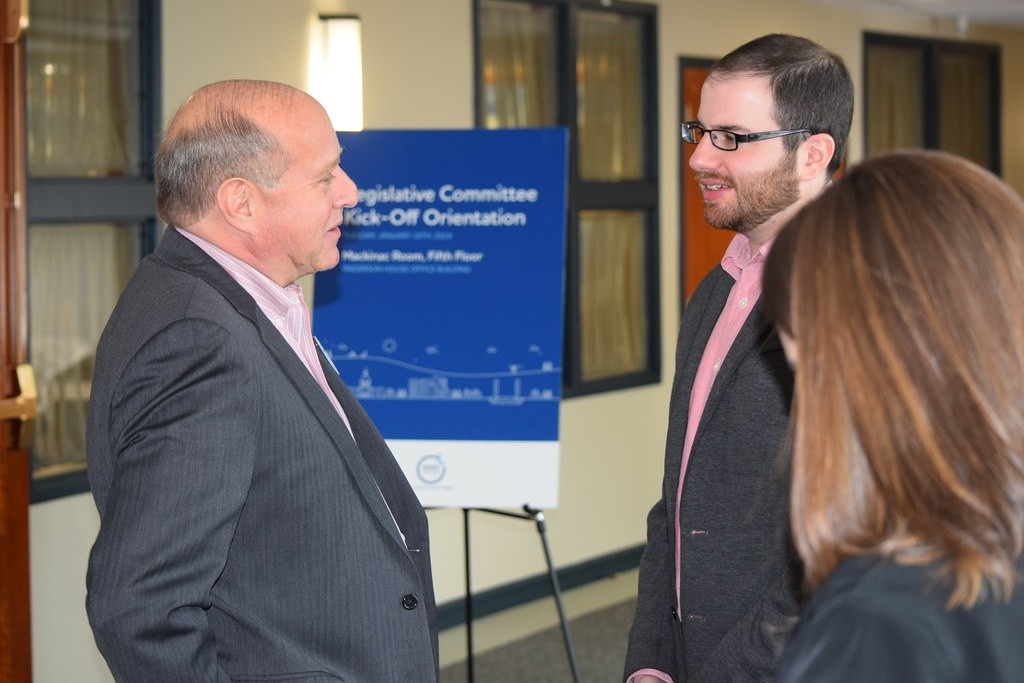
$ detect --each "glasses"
[680,121,812,151]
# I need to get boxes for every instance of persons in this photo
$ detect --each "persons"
[621,33,854,682]
[83,80,441,683]
[760,149,1024,683]
